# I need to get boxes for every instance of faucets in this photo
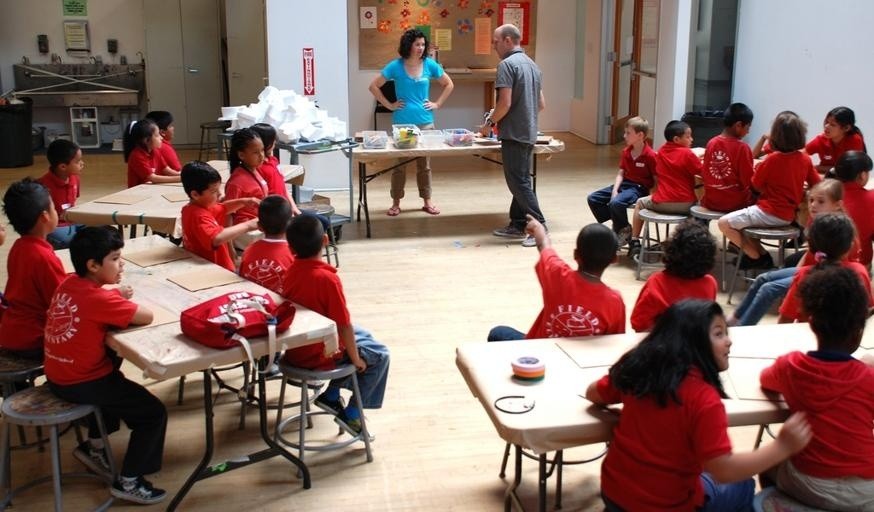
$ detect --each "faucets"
[89,56,100,64]
[135,50,144,64]
[53,55,63,65]
[20,55,31,66]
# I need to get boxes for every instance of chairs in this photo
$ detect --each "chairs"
[374,80,406,128]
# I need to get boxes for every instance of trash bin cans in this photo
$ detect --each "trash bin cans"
[683,111,726,148]
[0,97,33,168]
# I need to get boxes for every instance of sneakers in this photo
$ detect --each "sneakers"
[108,473,168,505]
[265,355,375,441]
[493,224,774,271]
[72,439,119,480]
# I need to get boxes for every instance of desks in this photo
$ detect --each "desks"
[455,324,873,512]
[68,161,305,239]
[342,131,563,237]
[53,235,336,512]
[430,73,499,130]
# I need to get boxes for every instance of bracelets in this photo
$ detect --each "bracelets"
[484,118,495,128]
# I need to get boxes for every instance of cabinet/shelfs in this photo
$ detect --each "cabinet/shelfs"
[226,0,267,135]
[143,1,226,151]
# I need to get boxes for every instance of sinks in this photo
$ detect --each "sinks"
[11,89,140,108]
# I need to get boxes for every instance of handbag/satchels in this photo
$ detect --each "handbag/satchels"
[181,292,295,350]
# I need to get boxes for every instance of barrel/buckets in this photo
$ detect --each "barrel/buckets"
[99,120,123,144]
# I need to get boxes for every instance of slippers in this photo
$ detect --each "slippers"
[387,208,401,216]
[423,206,440,214]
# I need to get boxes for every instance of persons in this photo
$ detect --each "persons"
[488,101,872,510]
[477,25,548,247]
[0,111,390,504]
[368,29,454,215]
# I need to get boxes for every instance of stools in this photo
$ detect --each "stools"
[753,486,834,512]
[1,350,86,460]
[274,366,372,481]
[198,121,235,162]
[629,207,800,313]
[239,355,317,429]
[1,388,123,512]
